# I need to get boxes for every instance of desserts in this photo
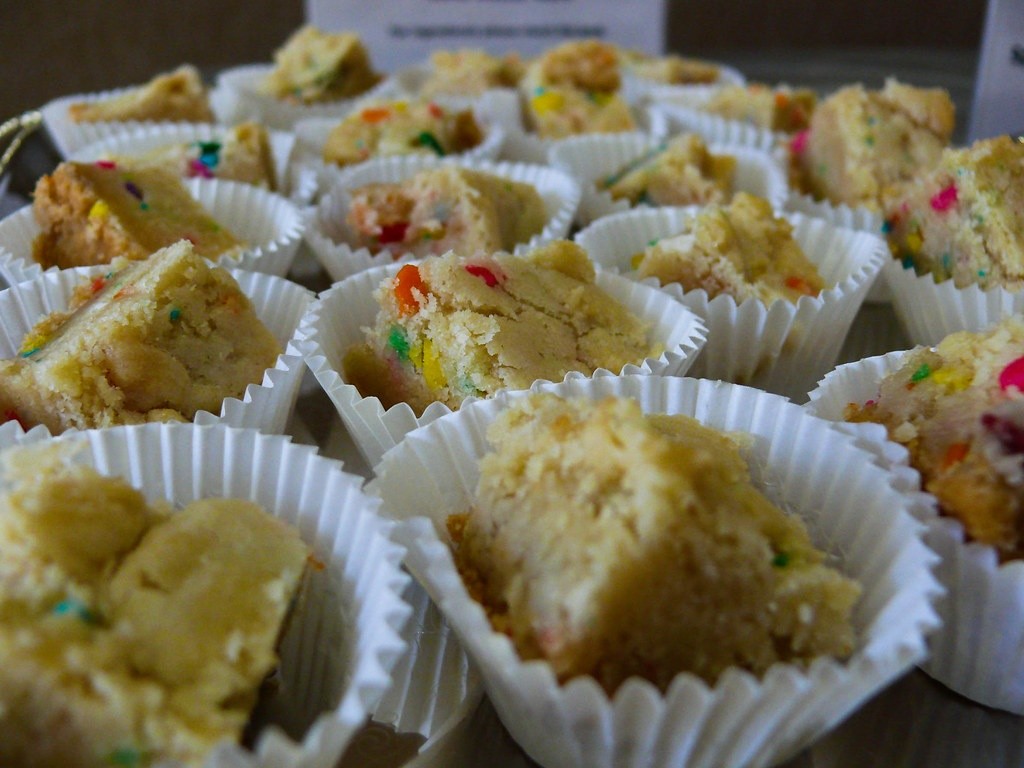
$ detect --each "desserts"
[0,21,1024,768]
